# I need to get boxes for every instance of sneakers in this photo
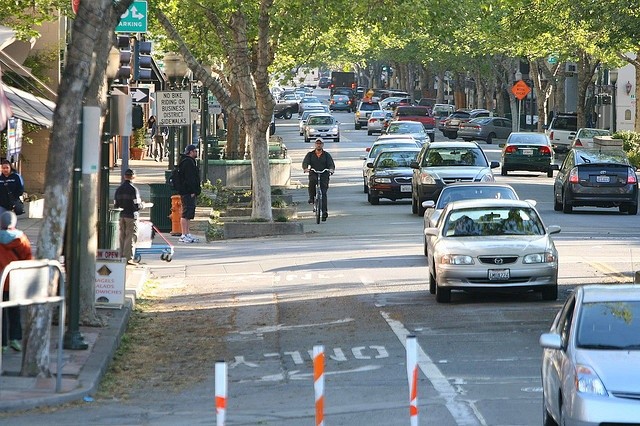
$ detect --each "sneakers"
[179,235,194,243]
[308,198,314,204]
[322,212,328,221]
[10,339,22,351]
[186,234,199,242]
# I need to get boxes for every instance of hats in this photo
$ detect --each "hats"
[315,137,324,143]
[125,169,134,174]
[180,145,195,155]
[0,211,17,228]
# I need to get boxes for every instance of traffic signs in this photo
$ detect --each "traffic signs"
[131,87,149,104]
[113,1,147,32]
[512,80,531,132]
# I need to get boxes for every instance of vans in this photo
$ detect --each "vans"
[381,97,411,107]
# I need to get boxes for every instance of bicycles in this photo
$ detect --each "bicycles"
[303,169,332,223]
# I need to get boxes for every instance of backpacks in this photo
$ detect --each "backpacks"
[169,157,196,191]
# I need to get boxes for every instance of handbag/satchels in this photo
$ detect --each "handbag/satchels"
[4,178,24,214]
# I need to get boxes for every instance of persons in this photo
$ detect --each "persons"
[143,117,169,161]
[0,159,26,215]
[171,144,201,244]
[302,138,335,222]
[112,168,145,265]
[1,210,32,353]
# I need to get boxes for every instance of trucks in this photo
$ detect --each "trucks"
[331,71,355,91]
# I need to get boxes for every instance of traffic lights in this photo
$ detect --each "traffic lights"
[118,36,130,76]
[329,83,335,96]
[133,33,152,80]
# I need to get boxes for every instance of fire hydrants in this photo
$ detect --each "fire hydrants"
[169,195,183,235]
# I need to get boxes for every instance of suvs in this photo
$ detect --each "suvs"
[410,143,499,216]
[396,105,434,142]
[355,102,382,129]
[570,127,610,148]
[542,112,597,152]
[445,109,489,138]
[319,78,329,88]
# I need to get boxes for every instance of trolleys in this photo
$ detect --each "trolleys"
[131,226,173,261]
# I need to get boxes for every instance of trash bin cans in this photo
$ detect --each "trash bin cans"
[149,183,180,238]
[199,136,218,156]
[109,208,124,251]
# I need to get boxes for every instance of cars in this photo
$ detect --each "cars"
[554,148,638,214]
[275,101,298,118]
[300,110,325,135]
[425,198,561,302]
[381,121,430,144]
[502,131,553,176]
[367,148,420,204]
[381,92,408,98]
[457,116,511,143]
[296,90,304,98]
[360,138,419,192]
[329,94,351,112]
[368,111,388,134]
[285,91,295,96]
[305,113,339,142]
[424,184,520,254]
[285,96,296,100]
[539,284,639,425]
[300,95,324,115]
